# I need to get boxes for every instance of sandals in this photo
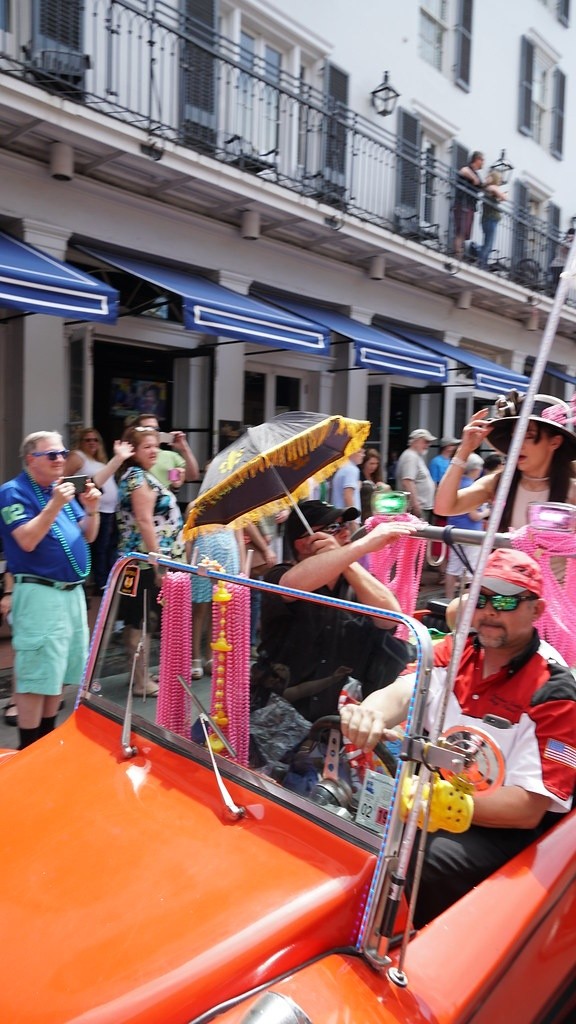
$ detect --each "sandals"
[204,657,214,676]
[191,659,205,679]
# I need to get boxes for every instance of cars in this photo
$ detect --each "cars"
[0,554,576,1024]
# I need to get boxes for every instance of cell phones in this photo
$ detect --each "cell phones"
[168,469,181,481]
[62,475,89,494]
[158,432,175,443]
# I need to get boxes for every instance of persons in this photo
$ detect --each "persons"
[0,413,503,747]
[137,385,164,416]
[191,499,403,797]
[433,388,576,631]
[550,228,575,299]
[337,548,576,929]
[480,169,509,266]
[453,151,485,261]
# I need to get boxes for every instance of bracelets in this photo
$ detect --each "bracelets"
[450,456,467,469]
[4,592,12,596]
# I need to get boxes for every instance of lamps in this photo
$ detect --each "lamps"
[457,292,474,309]
[50,140,74,181]
[490,149,512,182]
[372,72,399,116]
[241,210,262,242]
[526,313,540,331]
[368,255,384,281]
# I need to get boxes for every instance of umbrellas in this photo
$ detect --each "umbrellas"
[182,410,369,537]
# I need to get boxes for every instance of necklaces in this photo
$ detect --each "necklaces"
[521,474,549,480]
[25,470,91,576]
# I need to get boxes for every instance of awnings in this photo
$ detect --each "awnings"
[0,230,117,326]
[372,320,529,394]
[545,369,576,385]
[65,244,329,356]
[250,290,448,382]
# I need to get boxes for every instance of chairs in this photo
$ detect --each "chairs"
[224,136,278,178]
[302,172,347,206]
[484,249,510,277]
[516,259,545,291]
[22,36,89,99]
[393,204,439,246]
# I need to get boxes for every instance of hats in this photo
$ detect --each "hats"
[284,500,360,540]
[481,393,576,462]
[465,548,543,596]
[410,429,438,441]
[440,436,462,447]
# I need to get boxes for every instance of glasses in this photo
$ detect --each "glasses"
[130,427,154,436]
[85,437,98,443]
[299,521,350,540]
[475,593,537,610]
[154,427,160,432]
[35,448,70,461]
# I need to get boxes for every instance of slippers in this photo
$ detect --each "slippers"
[129,674,160,685]
[135,687,160,698]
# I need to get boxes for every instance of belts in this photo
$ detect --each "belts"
[14,576,80,592]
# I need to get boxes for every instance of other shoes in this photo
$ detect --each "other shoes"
[2,701,18,726]
